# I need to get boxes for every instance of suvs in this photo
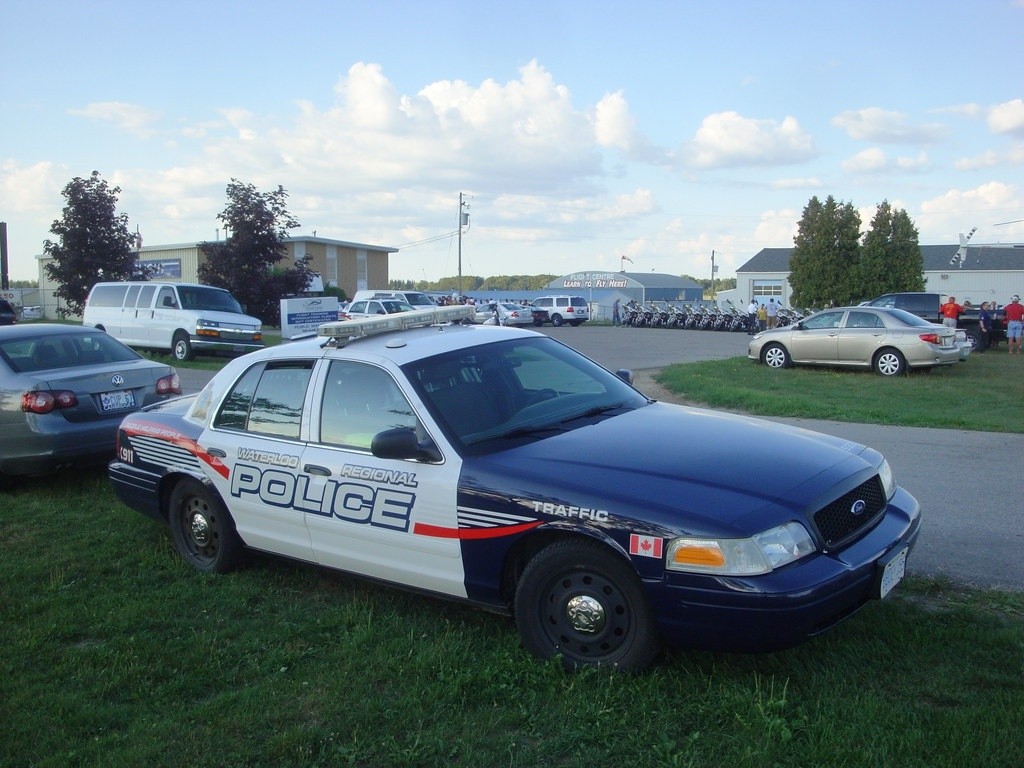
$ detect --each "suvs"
[523,294,589,326]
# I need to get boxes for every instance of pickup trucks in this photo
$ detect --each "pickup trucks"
[860,293,1024,352]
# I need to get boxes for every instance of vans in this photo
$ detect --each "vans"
[370,291,437,309]
[83,281,265,361]
[856,301,894,312]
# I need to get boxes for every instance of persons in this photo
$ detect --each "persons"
[758,303,768,331]
[990,301,996,310]
[432,295,528,307]
[1011,295,1020,300]
[963,301,971,308]
[747,300,757,335]
[978,301,990,351]
[763,299,779,330]
[1003,296,1024,354]
[612,299,623,327]
[940,297,965,329]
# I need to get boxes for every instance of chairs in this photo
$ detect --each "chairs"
[76,350,105,365]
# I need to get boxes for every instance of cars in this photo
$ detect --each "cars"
[0,298,16,325]
[115,304,926,677]
[0,322,182,481]
[472,301,535,326]
[344,299,417,320]
